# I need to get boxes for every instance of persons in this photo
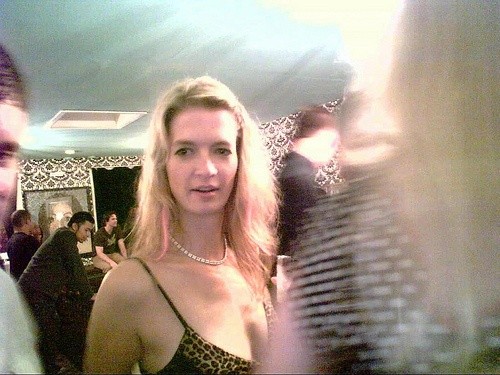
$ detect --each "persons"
[278,107,340,257]
[9,210,89,375]
[89,209,138,276]
[260,0,500,375]
[74,74,287,375]
[0,204,46,275]
[0,36,45,375]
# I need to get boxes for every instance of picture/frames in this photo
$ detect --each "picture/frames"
[23,186,97,259]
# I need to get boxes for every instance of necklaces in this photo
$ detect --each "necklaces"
[167,233,227,265]
